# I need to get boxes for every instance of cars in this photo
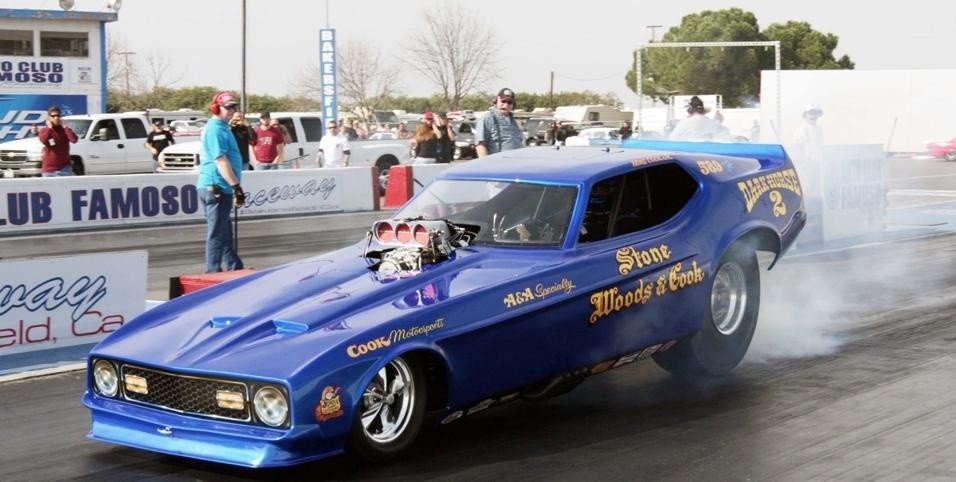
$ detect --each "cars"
[928,137,956,162]
[564,126,622,144]
[368,111,404,132]
[447,121,476,160]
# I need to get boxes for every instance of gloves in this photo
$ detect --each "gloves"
[231,184,245,205]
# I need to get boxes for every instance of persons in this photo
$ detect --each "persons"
[515,180,639,247]
[143,119,175,173]
[316,120,366,168]
[250,111,283,169]
[228,110,257,170]
[270,120,292,162]
[793,105,823,147]
[39,106,78,177]
[474,87,526,158]
[674,96,716,141]
[195,91,245,274]
[395,112,457,162]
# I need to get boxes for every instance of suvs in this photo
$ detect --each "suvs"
[0,112,159,177]
[521,118,575,147]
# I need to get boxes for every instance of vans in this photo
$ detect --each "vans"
[463,109,476,120]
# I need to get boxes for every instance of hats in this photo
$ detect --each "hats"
[151,117,163,125]
[259,111,270,119]
[210,93,240,107]
[424,112,433,119]
[498,88,515,102]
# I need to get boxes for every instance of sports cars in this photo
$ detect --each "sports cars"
[81,137,807,473]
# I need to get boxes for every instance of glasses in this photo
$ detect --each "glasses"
[328,126,337,129]
[50,114,60,117]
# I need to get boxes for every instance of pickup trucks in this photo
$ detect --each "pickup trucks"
[158,113,414,195]
